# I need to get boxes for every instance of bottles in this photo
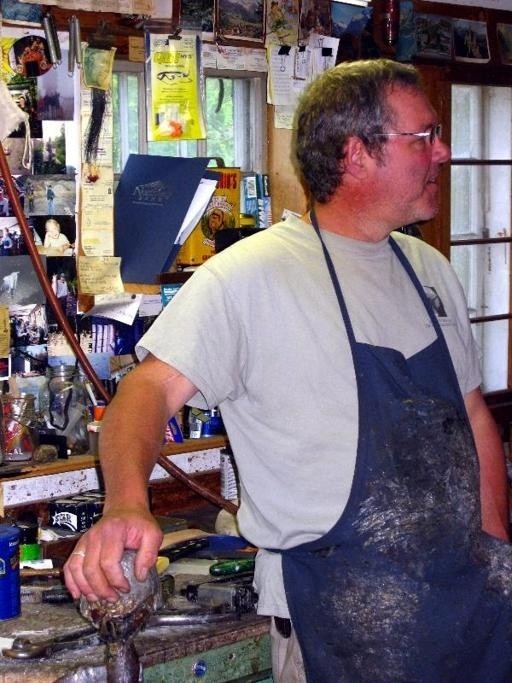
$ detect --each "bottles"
[39,366,88,455]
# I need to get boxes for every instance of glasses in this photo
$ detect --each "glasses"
[423,285,447,316]
[368,124,443,144]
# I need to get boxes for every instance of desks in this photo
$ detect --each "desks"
[3,504,275,682]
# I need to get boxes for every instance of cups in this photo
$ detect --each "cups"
[0,525,22,619]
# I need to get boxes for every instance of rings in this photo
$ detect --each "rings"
[72,550,86,558]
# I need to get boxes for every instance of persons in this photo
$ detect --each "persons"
[64,59,512,683]
[43,218,70,256]
